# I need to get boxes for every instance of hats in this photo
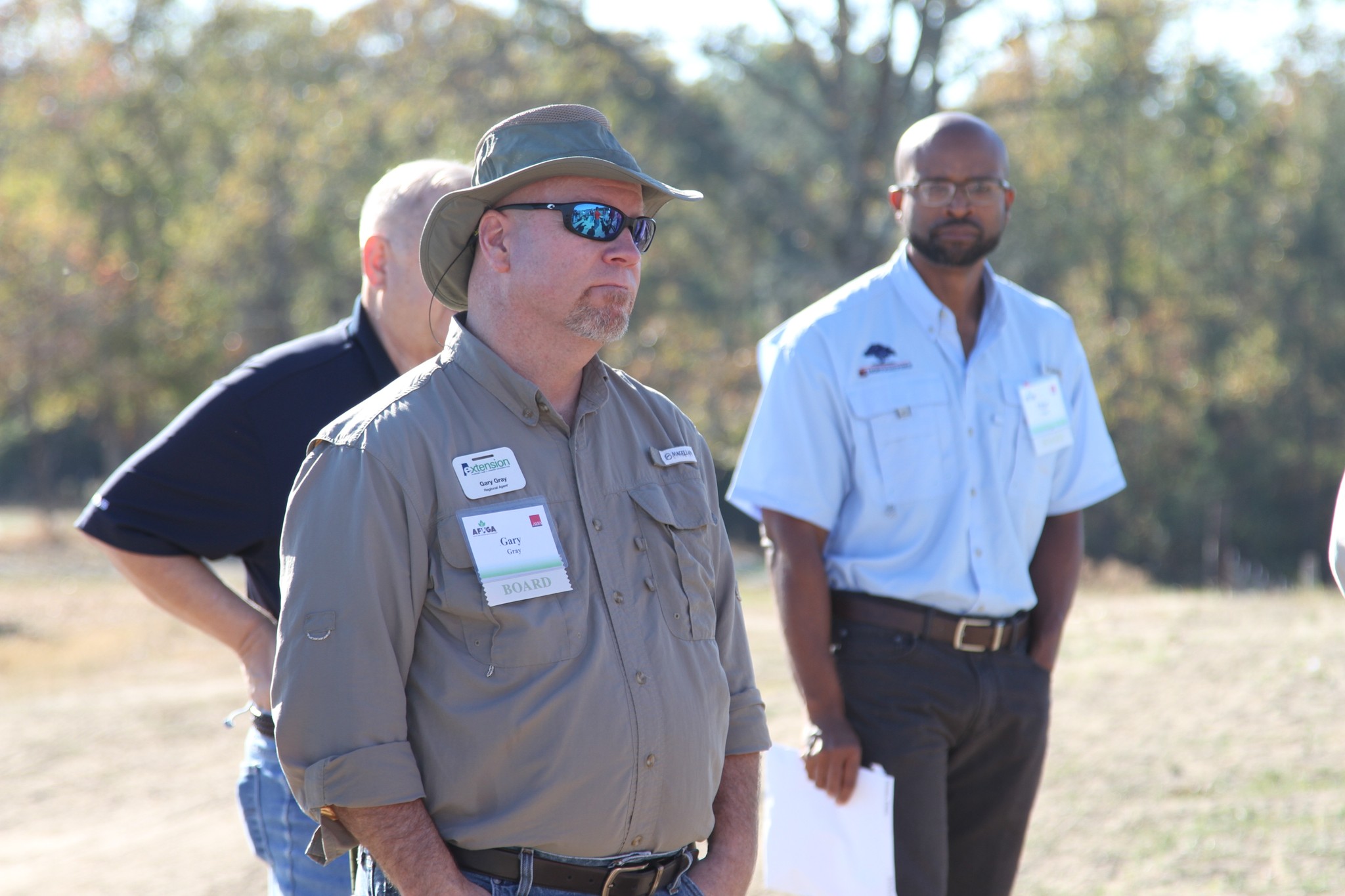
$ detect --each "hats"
[419,103,705,314]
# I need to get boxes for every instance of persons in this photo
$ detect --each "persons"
[725,111,1130,895]
[270,104,770,896]
[77,156,474,896]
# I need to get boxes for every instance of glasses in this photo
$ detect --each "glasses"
[465,200,658,255]
[900,174,1011,209]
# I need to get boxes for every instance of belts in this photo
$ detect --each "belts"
[829,588,1036,655]
[445,840,700,896]
[250,714,275,739]
